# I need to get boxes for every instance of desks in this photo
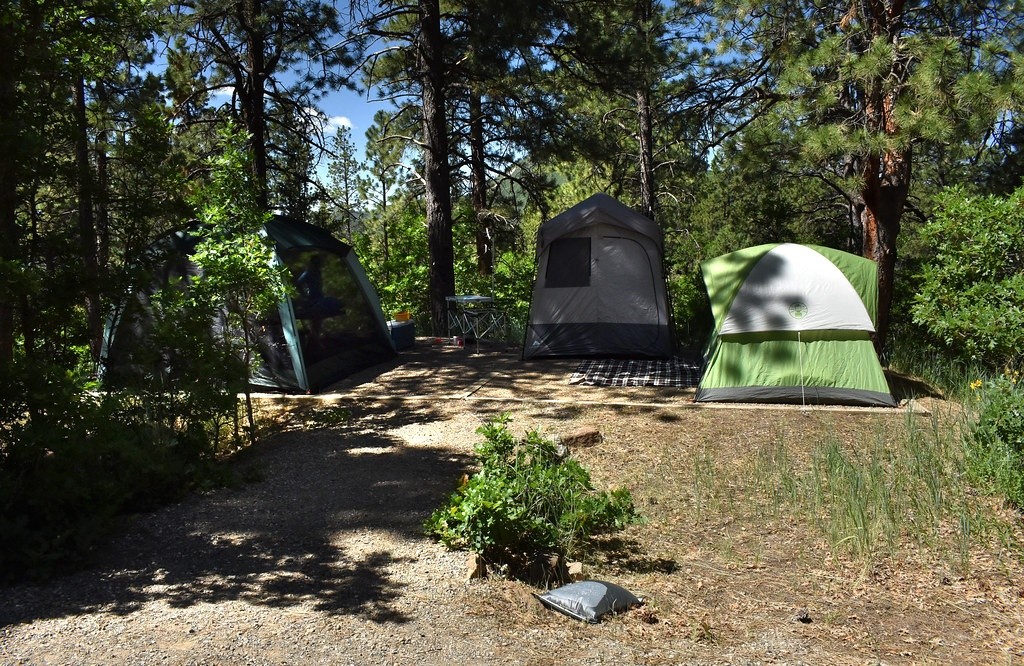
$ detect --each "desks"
[445,295,509,355]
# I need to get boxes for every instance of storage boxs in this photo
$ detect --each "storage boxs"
[387,318,416,353]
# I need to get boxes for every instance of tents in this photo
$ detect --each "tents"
[693,243,897,406]
[93,214,405,395]
[522,191,681,362]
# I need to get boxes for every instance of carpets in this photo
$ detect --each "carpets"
[570,358,702,388]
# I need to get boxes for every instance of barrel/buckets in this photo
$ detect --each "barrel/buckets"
[394,312,410,321]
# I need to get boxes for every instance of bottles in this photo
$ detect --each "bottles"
[453,335,462,346]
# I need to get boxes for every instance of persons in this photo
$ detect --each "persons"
[292,254,346,335]
